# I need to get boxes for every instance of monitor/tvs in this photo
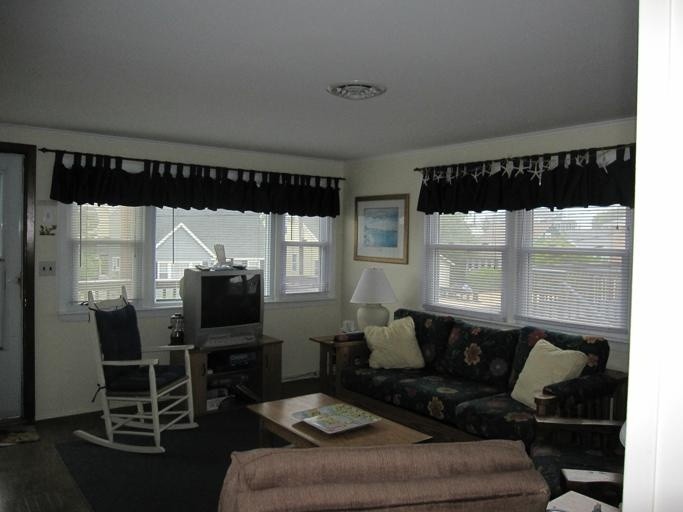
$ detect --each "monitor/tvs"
[180,270,264,348]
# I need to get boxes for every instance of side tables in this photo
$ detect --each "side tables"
[310,332,359,377]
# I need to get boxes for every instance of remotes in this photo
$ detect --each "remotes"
[196,265,210,271]
[233,265,246,269]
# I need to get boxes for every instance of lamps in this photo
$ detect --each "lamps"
[349,269,397,330]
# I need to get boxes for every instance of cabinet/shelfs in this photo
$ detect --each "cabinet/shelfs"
[165,336,284,420]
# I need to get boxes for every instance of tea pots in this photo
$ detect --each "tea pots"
[166,312,185,345]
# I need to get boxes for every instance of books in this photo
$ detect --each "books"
[233,378,259,401]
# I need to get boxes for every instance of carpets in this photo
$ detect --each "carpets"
[0,424,43,448]
[0,440,94,512]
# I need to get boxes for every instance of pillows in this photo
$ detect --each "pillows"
[435,318,518,394]
[363,316,424,369]
[511,341,587,408]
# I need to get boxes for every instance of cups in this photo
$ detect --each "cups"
[340,318,355,331]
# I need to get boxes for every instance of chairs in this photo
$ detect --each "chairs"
[70,287,198,454]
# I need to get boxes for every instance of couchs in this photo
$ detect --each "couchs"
[334,309,627,443]
[217,440,550,511]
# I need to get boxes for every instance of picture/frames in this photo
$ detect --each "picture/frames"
[354,192,409,265]
